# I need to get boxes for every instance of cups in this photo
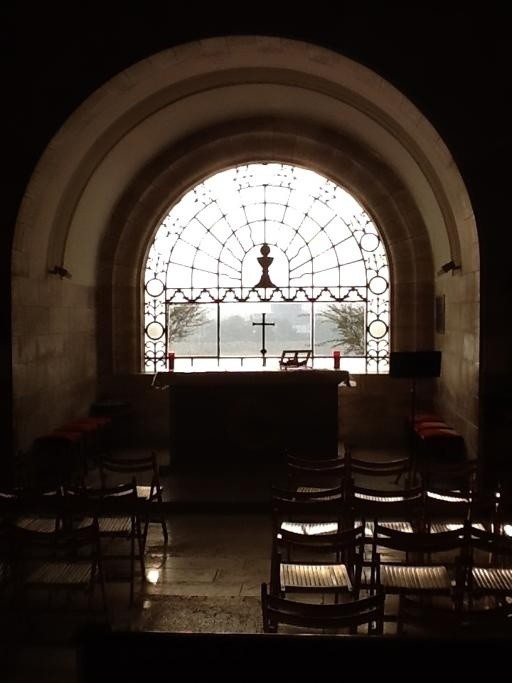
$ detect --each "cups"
[168,353,175,369]
[333,352,341,369]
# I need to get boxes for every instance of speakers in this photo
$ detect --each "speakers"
[390,351,441,377]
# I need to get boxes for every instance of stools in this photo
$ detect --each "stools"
[407,413,465,459]
[36,415,113,476]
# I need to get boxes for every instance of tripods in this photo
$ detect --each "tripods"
[395,378,426,488]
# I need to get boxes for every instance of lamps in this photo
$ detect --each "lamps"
[47,267,72,278]
[437,261,460,275]
